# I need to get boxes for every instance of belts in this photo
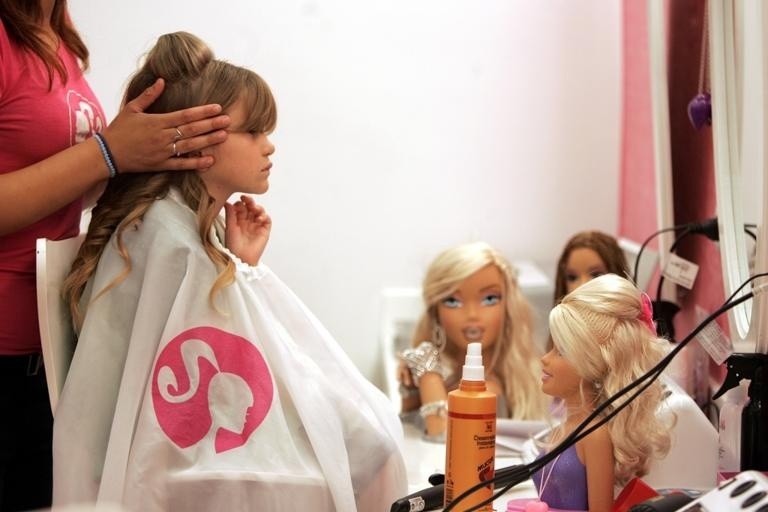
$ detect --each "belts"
[0,354,47,379]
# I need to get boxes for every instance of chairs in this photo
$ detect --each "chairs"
[33,228,86,421]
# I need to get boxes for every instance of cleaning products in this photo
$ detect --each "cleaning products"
[712,354,768,473]
[445,343,496,512]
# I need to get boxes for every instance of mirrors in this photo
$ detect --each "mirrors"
[703,0,767,359]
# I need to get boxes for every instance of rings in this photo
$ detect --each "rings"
[171,142,180,158]
[174,127,184,138]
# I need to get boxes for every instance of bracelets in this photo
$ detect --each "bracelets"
[93,133,121,179]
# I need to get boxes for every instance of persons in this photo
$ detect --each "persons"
[529,269,679,512]
[542,230,636,353]
[62,27,278,325]
[393,230,551,440]
[0,0,230,510]
